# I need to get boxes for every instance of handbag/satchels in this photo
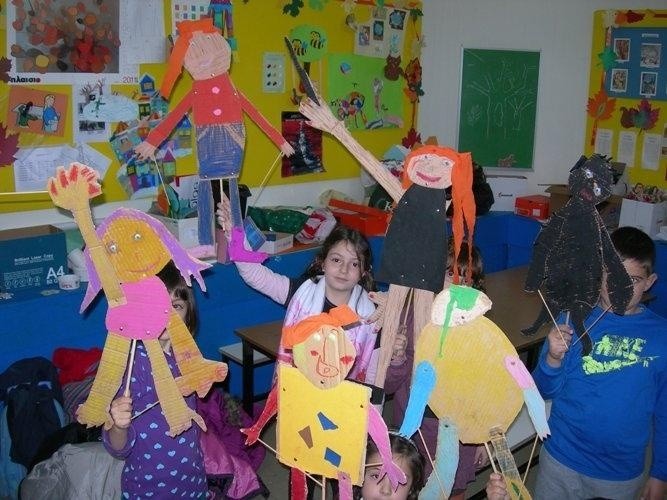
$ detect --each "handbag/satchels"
[0,358,66,466]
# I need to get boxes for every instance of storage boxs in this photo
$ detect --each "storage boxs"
[515,195,551,221]
[250,232,294,254]
[547,183,573,216]
[147,214,199,248]
[617,196,666,238]
[328,198,387,236]
[0,225,69,306]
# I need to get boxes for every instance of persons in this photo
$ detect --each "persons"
[217,193,379,499]
[354,427,424,500]
[382,235,485,500]
[101,268,214,499]
[532,226,666,499]
[484,472,523,500]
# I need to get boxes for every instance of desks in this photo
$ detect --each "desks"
[233,266,659,500]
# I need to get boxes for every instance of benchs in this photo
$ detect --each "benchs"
[463,399,551,500]
[219,341,274,419]
